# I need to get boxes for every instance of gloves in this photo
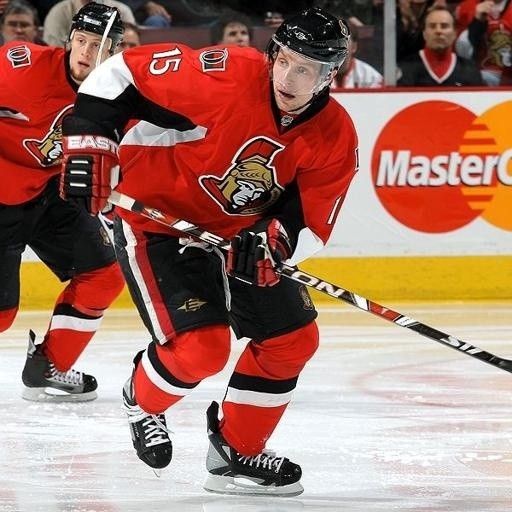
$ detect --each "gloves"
[58,112,121,218]
[224,217,292,288]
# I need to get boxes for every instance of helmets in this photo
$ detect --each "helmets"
[65,1,124,55]
[264,8,350,97]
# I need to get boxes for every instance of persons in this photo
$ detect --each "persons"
[0,3,130,395]
[44,0,140,52]
[134,0,216,28]
[210,8,255,47]
[60,7,361,485]
[328,24,384,90]
[396,0,512,89]
[0,0,38,43]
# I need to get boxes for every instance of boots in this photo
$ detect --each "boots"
[21,327,97,394]
[206,401,301,486]
[123,347,173,466]
